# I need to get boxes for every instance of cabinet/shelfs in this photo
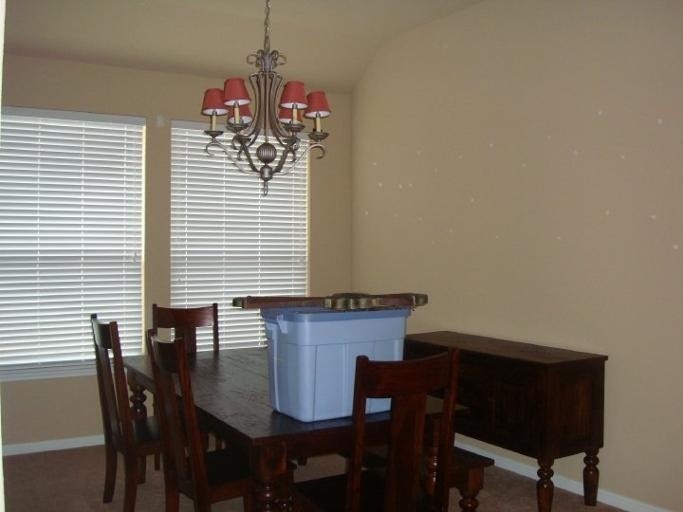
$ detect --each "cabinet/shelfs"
[407,332,607,511]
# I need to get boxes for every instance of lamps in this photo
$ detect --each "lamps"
[202,1,331,194]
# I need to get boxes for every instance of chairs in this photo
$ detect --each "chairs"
[150,304,220,352]
[90,313,224,512]
[294,348,480,511]
[146,330,297,512]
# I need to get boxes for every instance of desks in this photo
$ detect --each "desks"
[114,346,470,512]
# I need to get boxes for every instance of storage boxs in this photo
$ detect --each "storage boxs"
[258,308,412,423]
